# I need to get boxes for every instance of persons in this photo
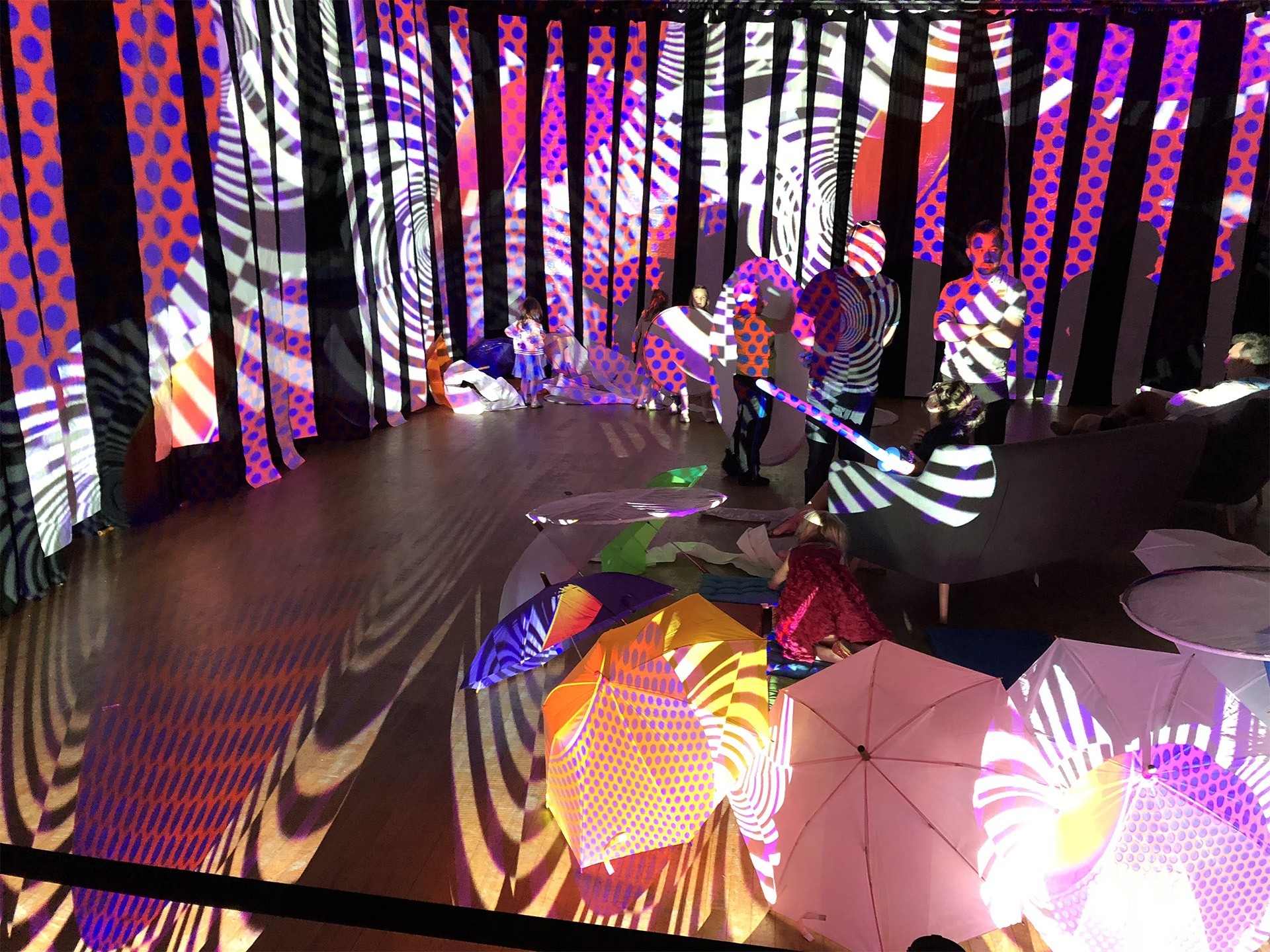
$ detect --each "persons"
[767,380,988,536]
[767,509,892,663]
[933,220,1028,445]
[721,278,776,487]
[545,325,588,404]
[790,220,901,505]
[504,296,547,408]
[630,284,716,423]
[1051,332,1270,436]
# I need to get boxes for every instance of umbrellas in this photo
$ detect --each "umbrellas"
[425,332,490,409]
[458,572,675,698]
[541,593,773,873]
[588,343,636,399]
[467,337,516,379]
[498,465,711,625]
[1131,529,1270,728]
[726,636,1270,952]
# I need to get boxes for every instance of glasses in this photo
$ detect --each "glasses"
[849,220,881,244]
[926,394,943,413]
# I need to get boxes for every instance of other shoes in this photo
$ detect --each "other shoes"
[653,404,664,410]
[739,470,770,486]
[636,403,646,409]
[680,414,690,423]
[701,414,715,422]
[722,448,744,478]
[767,516,799,538]
[525,397,543,408]
